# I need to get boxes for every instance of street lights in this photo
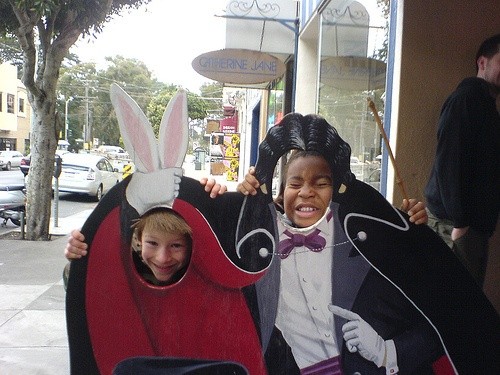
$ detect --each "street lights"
[64,96,74,141]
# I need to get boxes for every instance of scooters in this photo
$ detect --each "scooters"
[0,152,62,227]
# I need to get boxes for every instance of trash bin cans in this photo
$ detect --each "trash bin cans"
[195,162,201,170]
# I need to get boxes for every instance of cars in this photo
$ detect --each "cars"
[56,138,127,160]
[0,150,26,171]
[52,153,119,202]
[20,153,31,178]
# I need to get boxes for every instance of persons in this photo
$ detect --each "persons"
[237,149,428,229]
[426,34,500,291]
[62,178,227,292]
